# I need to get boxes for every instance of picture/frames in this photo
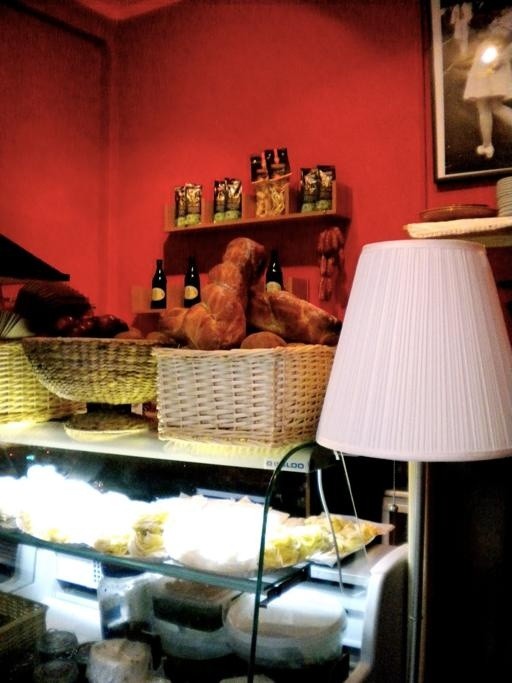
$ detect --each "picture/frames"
[425,0,512,190]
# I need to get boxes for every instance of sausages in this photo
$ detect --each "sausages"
[318,228,345,301]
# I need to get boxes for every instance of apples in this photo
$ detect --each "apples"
[57,314,127,337]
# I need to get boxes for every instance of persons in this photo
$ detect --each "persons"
[464,0,511,157]
[448,0,473,45]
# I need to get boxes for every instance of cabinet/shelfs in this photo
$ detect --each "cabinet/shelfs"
[0,415,405,683]
[131,180,352,318]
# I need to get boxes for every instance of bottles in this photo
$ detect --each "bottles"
[150,259,168,309]
[266,248,283,295]
[184,254,202,309]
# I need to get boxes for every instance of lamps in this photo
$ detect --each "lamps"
[312,231,511,683]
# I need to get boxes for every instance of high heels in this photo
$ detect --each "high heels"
[477,143,495,159]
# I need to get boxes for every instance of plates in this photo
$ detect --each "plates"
[494,175,511,217]
[419,204,497,221]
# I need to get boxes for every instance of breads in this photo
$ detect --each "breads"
[146,237,342,350]
[115,331,144,338]
